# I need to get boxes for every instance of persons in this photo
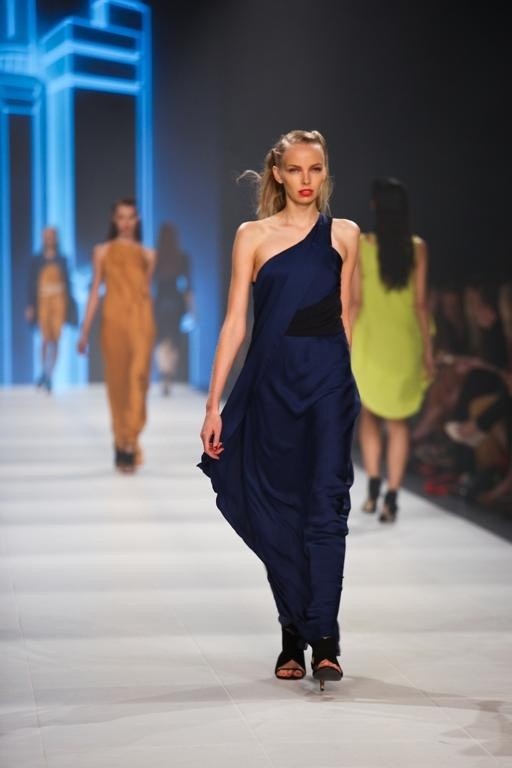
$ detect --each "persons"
[149,220,195,398]
[353,277,510,508]
[77,201,157,475]
[27,228,76,392]
[196,127,360,692]
[348,177,433,525]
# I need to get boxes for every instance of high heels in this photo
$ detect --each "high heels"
[379,491,398,524]
[363,475,381,513]
[311,653,343,691]
[274,631,306,680]
